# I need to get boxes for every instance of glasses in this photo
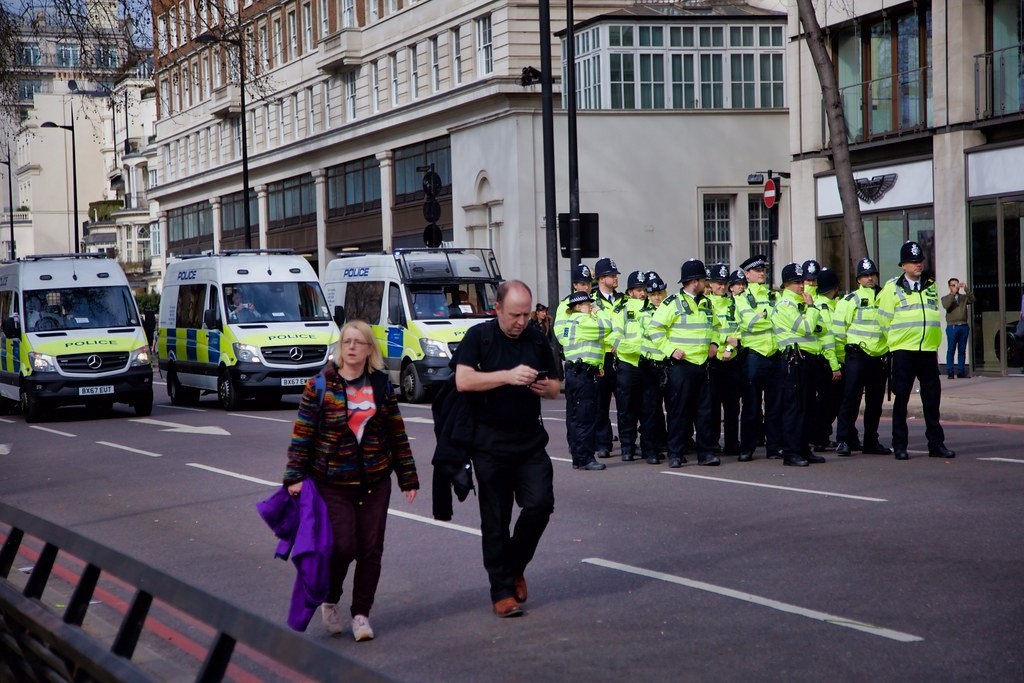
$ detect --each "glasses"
[340,338,373,348]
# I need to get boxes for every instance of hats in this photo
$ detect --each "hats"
[645,279,668,293]
[740,255,767,272]
[568,290,595,308]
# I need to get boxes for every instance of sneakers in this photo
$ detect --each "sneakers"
[321,602,345,634]
[351,614,374,642]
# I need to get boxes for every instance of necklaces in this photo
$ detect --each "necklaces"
[339,372,366,393]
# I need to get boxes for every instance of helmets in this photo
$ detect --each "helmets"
[855,258,881,285]
[678,258,708,283]
[779,263,807,289]
[705,264,730,282]
[727,269,748,293]
[898,240,925,267]
[570,264,593,283]
[626,270,647,295]
[595,258,621,279]
[645,272,658,283]
[801,260,820,280]
[816,266,839,293]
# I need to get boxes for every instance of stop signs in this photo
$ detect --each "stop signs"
[763,179,776,208]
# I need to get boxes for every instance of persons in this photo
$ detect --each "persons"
[553,241,956,470]
[150,323,159,354]
[528,303,565,393]
[941,278,976,379]
[283,320,419,641]
[448,279,564,617]
[26,297,46,332]
[229,289,261,321]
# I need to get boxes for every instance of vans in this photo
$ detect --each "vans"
[321,246,507,404]
[0,253,156,425]
[156,249,344,412]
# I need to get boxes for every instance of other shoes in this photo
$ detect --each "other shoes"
[947,374,955,379]
[956,374,972,379]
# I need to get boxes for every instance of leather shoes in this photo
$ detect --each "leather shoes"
[513,573,528,603]
[570,434,959,471]
[493,598,522,617]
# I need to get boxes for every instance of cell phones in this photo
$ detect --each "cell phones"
[532,370,549,384]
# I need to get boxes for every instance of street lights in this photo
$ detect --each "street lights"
[195,4,252,249]
[40,102,79,258]
